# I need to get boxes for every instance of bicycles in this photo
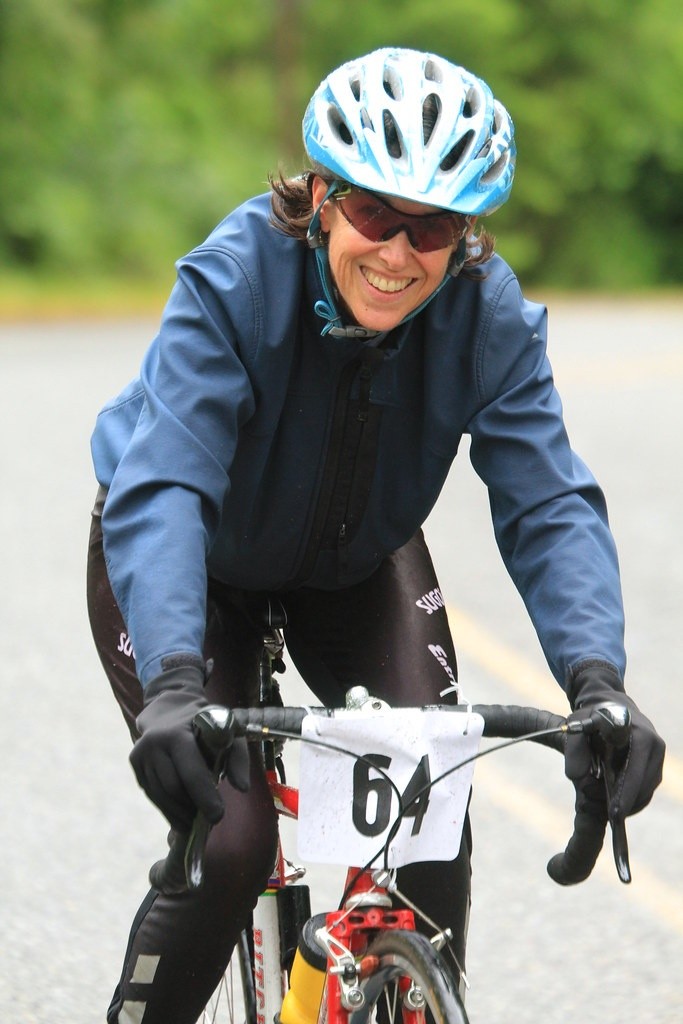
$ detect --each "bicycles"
[142,597,645,1024]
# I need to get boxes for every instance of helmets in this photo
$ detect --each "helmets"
[303,47,516,218]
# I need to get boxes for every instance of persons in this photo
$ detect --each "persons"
[86,46,666,1024]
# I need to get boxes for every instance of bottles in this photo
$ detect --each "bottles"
[278,912,328,1024]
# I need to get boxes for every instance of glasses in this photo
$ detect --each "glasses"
[323,179,472,254]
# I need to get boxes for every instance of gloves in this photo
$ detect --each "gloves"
[563,659,666,823]
[129,665,251,835]
